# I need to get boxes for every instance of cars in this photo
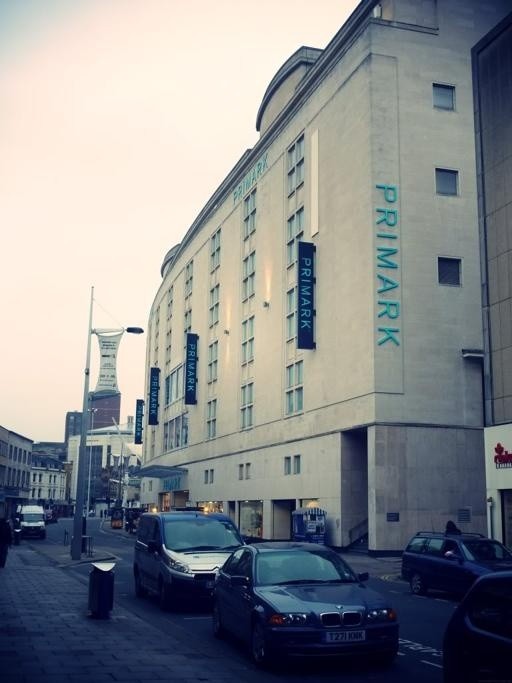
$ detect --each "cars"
[46,510,57,523]
[443,572,512,683]
[209,540,402,670]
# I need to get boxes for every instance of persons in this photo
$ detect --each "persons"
[1,516,13,568]
[438,519,463,555]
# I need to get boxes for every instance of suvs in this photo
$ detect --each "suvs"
[401,532,512,602]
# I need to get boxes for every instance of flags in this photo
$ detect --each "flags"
[93,329,125,393]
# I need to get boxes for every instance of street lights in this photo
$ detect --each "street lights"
[72,286,144,561]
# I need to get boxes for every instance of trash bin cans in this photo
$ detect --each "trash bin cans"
[88,562,116,620]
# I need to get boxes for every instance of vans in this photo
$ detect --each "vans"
[19,505,46,540]
[133,510,248,616]
